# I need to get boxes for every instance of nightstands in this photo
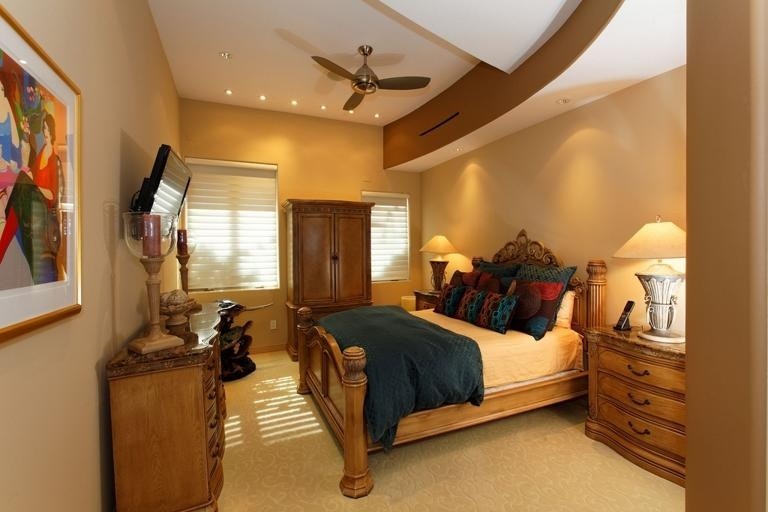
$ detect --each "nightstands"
[582,323,688,488]
[413,286,440,309]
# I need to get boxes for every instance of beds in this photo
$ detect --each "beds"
[294,226,606,501]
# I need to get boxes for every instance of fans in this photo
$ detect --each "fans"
[311,41,432,110]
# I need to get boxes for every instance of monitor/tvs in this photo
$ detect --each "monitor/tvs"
[132,144,192,240]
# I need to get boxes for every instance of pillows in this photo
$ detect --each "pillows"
[433,259,579,343]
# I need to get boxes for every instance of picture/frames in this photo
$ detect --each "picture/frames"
[0,3,87,344]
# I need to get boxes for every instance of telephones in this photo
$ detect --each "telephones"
[613,301,635,331]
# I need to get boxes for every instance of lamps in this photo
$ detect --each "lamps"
[416,234,454,288]
[613,221,687,346]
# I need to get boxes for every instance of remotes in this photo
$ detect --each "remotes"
[615,301,635,329]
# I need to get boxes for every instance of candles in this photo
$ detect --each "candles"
[176,230,188,255]
[140,213,163,257]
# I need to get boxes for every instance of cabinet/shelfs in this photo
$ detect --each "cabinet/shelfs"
[106,301,227,512]
[283,197,376,361]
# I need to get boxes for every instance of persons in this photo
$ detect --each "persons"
[0,113,58,265]
[1,80,20,168]
